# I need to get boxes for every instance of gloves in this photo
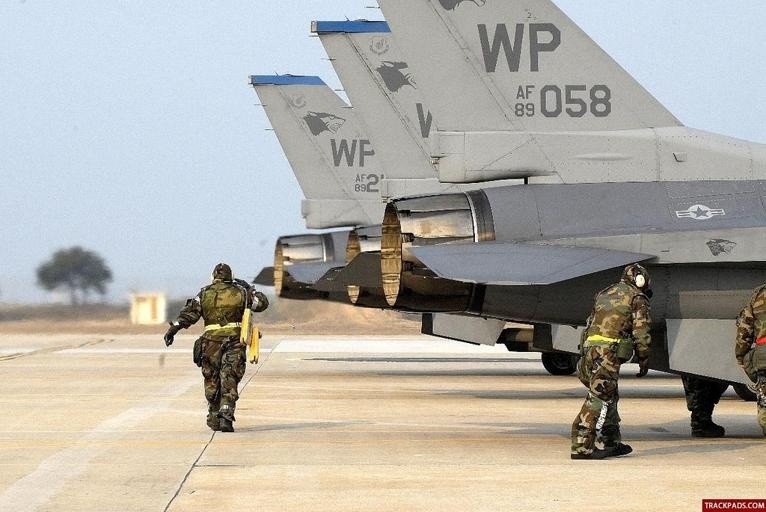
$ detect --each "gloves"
[634,348,651,379]
[162,320,180,348]
[232,277,256,292]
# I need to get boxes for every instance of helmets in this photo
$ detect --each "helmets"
[620,262,654,299]
[212,262,233,281]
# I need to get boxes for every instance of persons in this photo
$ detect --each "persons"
[162,259,268,434]
[734,278,765,440]
[568,262,655,460]
[677,373,729,440]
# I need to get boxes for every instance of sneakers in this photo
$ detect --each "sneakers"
[206,411,235,433]
[569,441,633,460]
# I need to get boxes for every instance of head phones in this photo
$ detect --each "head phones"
[630,262,646,289]
